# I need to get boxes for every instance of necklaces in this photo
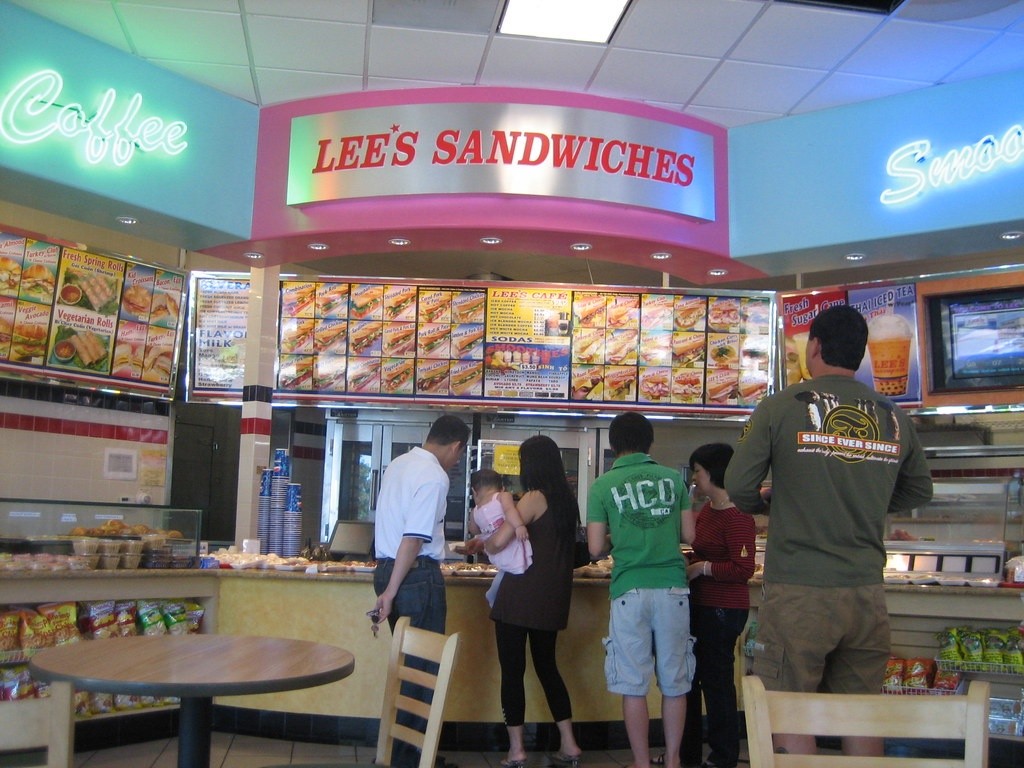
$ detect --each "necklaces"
[711,497,728,509]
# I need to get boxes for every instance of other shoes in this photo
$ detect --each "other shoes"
[650,753,665,764]
[702,760,718,768]
[435,752,459,768]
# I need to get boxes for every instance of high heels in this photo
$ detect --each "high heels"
[551,751,582,768]
[499,752,528,768]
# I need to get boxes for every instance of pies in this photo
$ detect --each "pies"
[70,330,107,366]
[79,275,114,312]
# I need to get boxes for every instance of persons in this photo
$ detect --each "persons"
[586,412,695,768]
[468,469,533,609]
[484,436,583,768]
[724,305,934,756]
[650,443,756,768]
[373,415,470,768]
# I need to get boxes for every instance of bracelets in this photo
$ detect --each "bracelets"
[703,560,709,576]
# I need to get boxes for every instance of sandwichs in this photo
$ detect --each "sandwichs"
[113,343,171,379]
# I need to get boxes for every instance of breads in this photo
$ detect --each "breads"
[71,520,184,538]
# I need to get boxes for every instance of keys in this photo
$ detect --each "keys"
[371,622,379,637]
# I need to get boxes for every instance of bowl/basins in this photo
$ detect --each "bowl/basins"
[60,283,83,305]
[143,534,197,568]
[70,539,146,569]
[54,340,77,362]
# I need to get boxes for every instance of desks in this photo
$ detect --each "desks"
[28,633,356,768]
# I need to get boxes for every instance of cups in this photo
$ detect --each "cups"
[495,351,503,361]
[513,352,521,361]
[503,351,512,362]
[256,448,303,557]
[541,353,550,365]
[532,353,540,364]
[522,354,530,364]
[792,332,812,380]
[866,314,914,395]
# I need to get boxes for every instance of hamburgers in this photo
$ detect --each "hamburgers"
[571,301,767,405]
[0,257,56,357]
[278,287,485,398]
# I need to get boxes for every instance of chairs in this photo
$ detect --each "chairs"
[741,673,991,767]
[260,616,464,768]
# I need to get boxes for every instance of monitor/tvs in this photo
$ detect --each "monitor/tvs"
[327,520,374,563]
[930,288,1024,390]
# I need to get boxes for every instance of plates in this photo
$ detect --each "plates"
[884,576,1001,588]
[572,568,612,578]
[440,567,498,577]
[231,561,378,571]
